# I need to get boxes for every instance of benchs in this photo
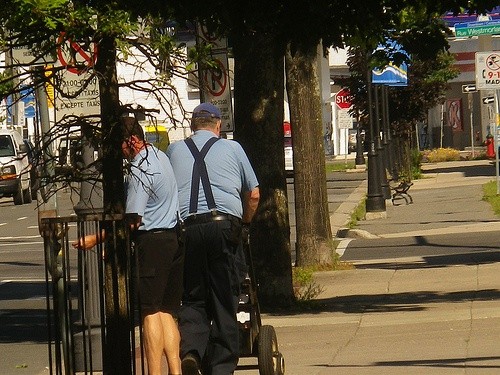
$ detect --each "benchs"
[388,176,413,205]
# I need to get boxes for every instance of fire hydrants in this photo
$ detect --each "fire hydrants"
[485,133,496,159]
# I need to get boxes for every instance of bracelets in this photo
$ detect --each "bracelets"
[242,223,251,226]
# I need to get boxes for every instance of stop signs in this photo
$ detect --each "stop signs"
[335,88,353,109]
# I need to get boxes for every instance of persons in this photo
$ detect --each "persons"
[166,102,260,375]
[72,117,183,375]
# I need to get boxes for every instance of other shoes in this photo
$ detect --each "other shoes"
[181,353,200,375]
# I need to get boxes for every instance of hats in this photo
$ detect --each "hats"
[192,102,221,119]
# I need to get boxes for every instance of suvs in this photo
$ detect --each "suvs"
[0,129,39,206]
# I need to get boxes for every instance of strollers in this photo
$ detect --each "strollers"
[198,226,286,375]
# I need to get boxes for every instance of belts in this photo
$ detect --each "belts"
[183,215,230,225]
